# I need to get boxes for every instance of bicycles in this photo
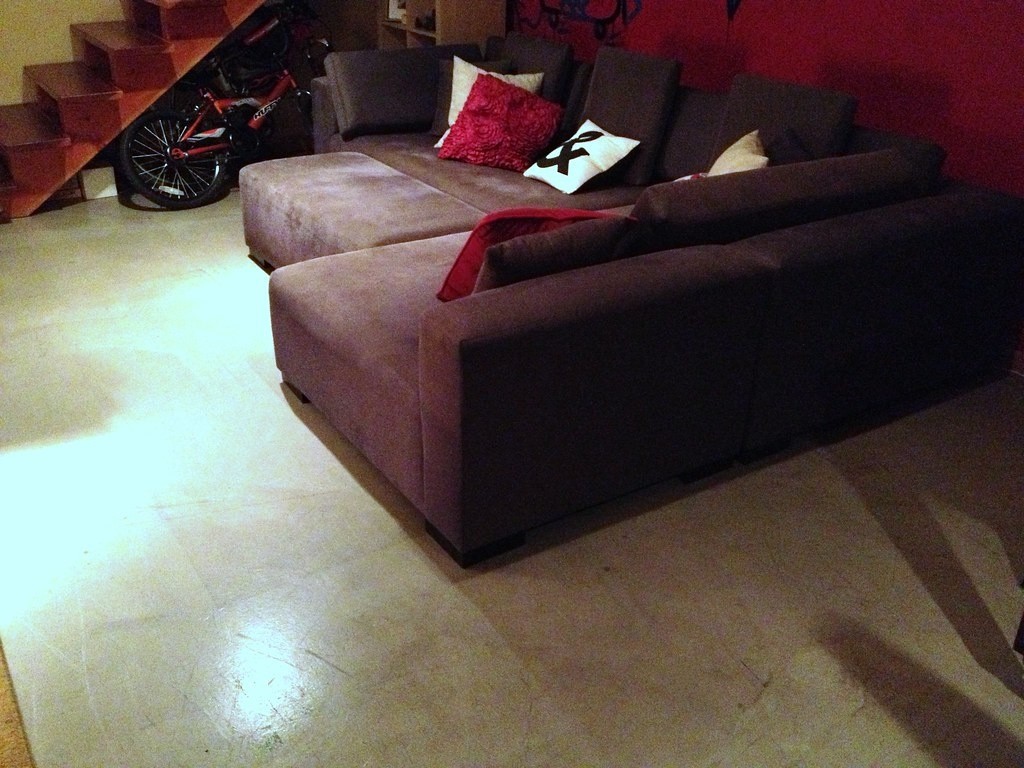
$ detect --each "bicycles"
[119,1,335,211]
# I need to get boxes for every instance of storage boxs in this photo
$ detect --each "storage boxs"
[80,162,117,200]
[53,182,80,201]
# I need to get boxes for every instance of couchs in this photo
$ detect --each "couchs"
[237,44,1023,563]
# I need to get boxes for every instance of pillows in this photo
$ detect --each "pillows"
[524,120,639,194]
[434,55,544,151]
[438,75,563,174]
[706,131,769,176]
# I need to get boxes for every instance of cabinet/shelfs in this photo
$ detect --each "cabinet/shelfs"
[376,1,505,51]
